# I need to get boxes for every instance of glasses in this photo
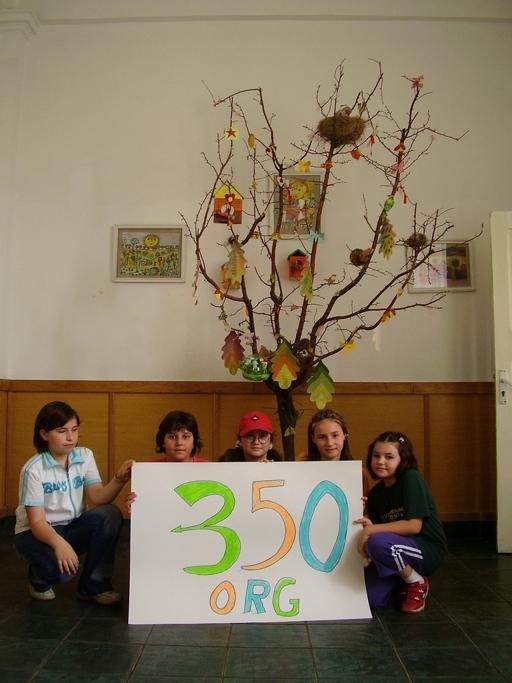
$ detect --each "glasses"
[242,433,270,443]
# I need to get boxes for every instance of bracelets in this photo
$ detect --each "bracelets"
[114,474,128,482]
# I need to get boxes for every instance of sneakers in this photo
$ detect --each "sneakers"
[73,591,121,605]
[400,576,430,613]
[29,585,55,600]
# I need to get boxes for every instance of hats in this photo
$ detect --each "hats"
[239,411,273,436]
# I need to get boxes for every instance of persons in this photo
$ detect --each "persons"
[217,410,282,462]
[294,410,370,513]
[352,429,447,615]
[125,409,210,515]
[10,399,135,605]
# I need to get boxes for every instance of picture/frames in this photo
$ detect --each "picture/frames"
[406,240,476,293]
[267,172,322,240]
[113,224,185,284]
